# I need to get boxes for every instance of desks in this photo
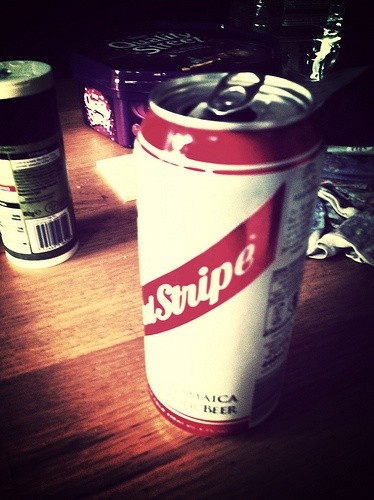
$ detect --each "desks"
[0,101,374,499]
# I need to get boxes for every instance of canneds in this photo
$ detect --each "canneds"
[0,60,79,271]
[131,70,330,439]
